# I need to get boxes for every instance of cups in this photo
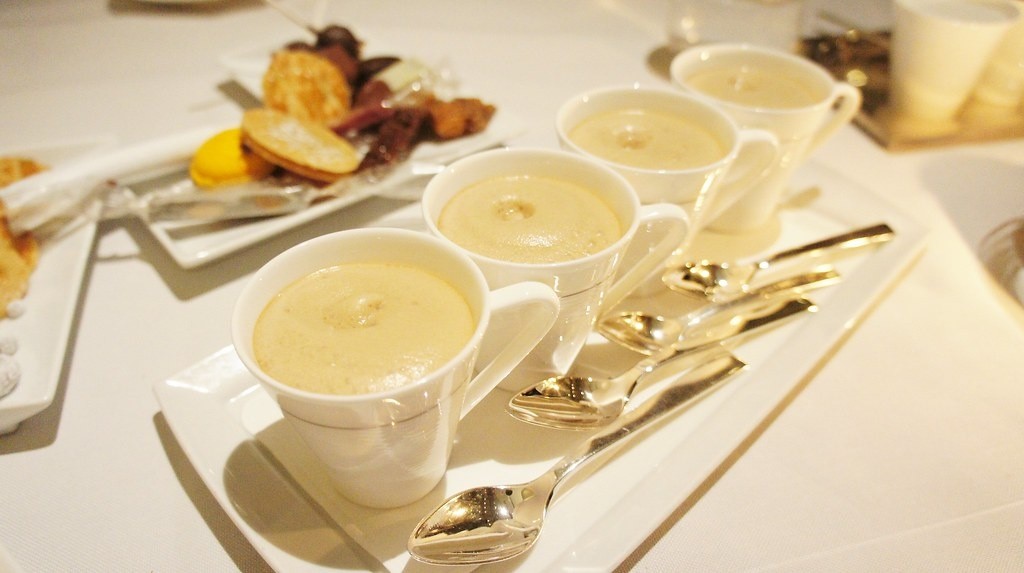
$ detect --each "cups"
[558,85,784,298]
[420,146,690,393]
[231,227,560,510]
[669,43,862,234]
[889,0,1024,120]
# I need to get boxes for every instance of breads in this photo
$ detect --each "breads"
[187,50,359,190]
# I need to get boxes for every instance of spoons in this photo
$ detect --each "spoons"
[504,297,814,432]
[406,352,745,565]
[596,270,840,357]
[660,224,896,301]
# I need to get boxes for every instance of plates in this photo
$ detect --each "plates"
[123,86,506,271]
[153,113,927,572]
[0,137,101,436]
